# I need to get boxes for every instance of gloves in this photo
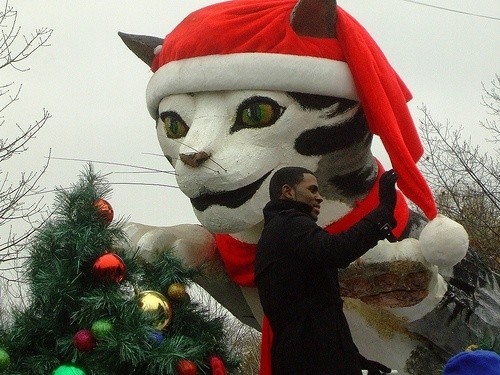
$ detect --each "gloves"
[376,165,400,221]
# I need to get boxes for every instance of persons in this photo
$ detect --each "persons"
[251,165,401,375]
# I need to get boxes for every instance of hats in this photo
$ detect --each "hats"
[437,345,500,375]
